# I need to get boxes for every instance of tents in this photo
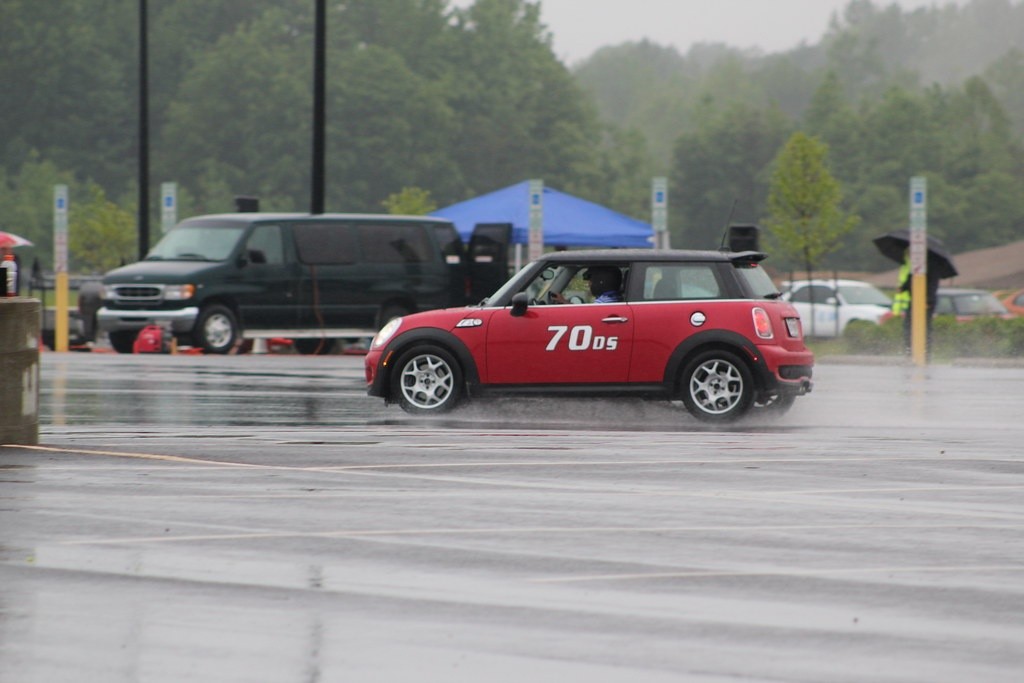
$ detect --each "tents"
[426,180,654,276]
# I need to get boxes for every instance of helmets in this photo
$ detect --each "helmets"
[583,265,622,296]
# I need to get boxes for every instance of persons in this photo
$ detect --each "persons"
[893,245,938,356]
[552,267,624,303]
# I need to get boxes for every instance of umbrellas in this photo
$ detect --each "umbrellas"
[872,228,959,279]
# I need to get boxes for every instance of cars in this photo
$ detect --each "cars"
[931,288,1014,326]
[777,279,906,334]
[363,246,817,422]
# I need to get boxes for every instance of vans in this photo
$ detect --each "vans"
[92,212,467,355]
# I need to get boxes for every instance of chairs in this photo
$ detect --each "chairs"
[620,270,678,300]
